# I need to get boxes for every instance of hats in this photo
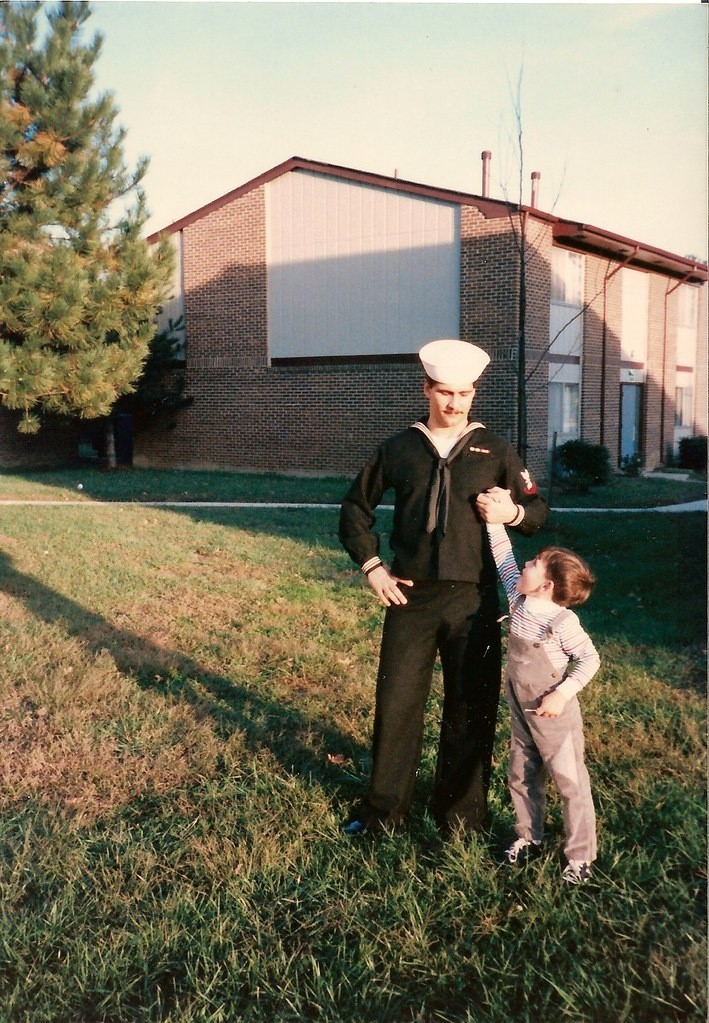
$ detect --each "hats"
[418,340,491,385]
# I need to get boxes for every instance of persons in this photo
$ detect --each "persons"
[338,340,552,836]
[485,488,601,883]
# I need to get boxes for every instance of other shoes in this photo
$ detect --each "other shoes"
[341,816,403,833]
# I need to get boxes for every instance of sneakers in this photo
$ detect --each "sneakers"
[558,860,589,886]
[501,839,543,865]
